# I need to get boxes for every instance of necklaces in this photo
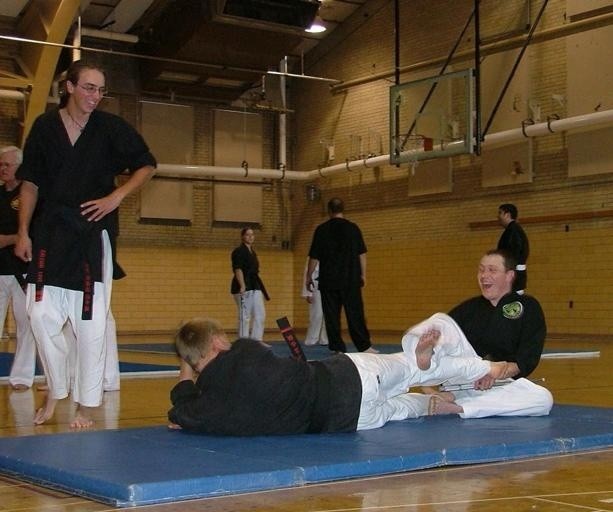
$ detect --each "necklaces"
[66,108,83,130]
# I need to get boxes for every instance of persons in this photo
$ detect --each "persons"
[166,316,509,437]
[0,146,37,392]
[301,255,329,347]
[12,60,159,431]
[497,204,529,295]
[63,305,121,395]
[400,248,554,421]
[306,198,380,355]
[230,227,272,349]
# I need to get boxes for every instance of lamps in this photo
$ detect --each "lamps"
[304,9,326,34]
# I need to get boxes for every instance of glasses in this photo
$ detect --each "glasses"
[76,81,109,95]
